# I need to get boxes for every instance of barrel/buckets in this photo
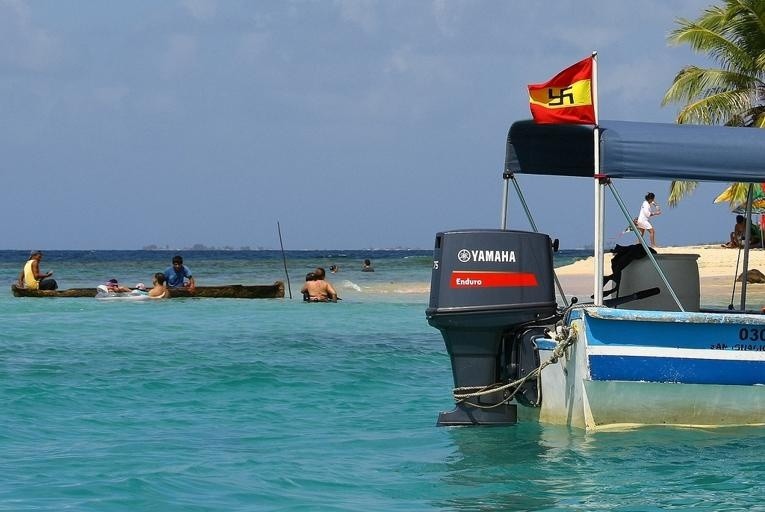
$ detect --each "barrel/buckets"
[610,252,701,313]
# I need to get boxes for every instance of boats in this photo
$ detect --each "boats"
[12,281,286,297]
[424,115,765,429]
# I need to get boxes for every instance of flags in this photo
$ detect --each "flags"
[526,55,596,127]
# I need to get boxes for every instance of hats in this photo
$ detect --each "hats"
[29,250,41,260]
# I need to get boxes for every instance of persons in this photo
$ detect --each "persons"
[96,272,170,299]
[300,264,339,303]
[721,214,761,249]
[18,249,58,291]
[163,254,195,294]
[361,259,374,272]
[637,193,662,248]
